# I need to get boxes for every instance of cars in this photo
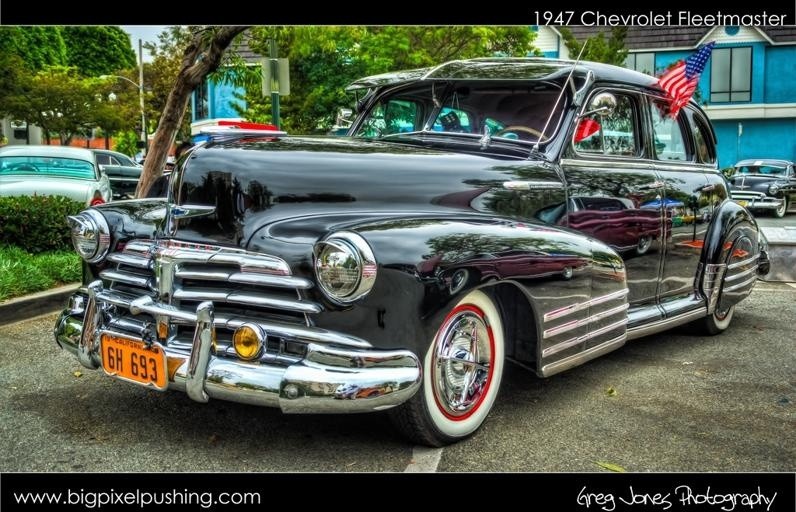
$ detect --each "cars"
[723,158,791,220]
[1,146,145,207]
[53,55,772,446]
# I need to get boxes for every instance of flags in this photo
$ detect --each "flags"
[654,40,715,119]
